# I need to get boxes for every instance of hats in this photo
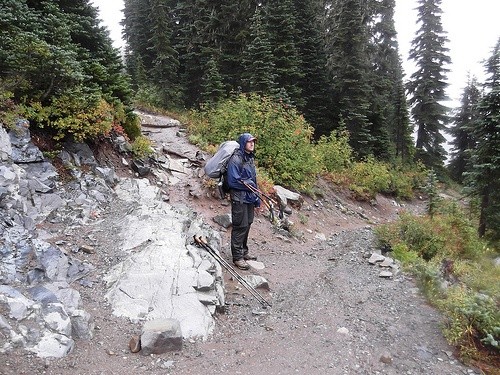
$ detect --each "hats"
[247,137,257,142]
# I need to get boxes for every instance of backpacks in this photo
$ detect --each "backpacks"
[205,141,240,178]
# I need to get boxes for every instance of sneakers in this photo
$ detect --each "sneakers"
[234,260,247,271]
[244,253,257,260]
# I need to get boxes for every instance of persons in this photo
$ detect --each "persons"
[226,133,261,271]
